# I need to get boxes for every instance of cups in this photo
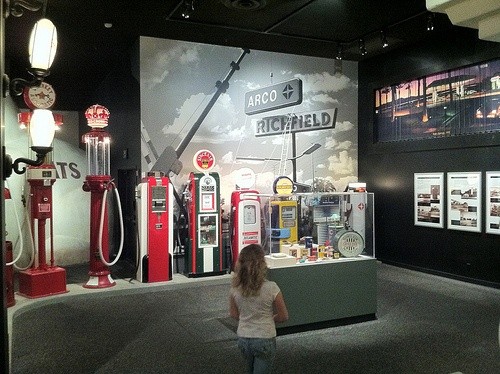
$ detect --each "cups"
[281,243,335,262]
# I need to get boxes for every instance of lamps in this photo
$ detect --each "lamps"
[2,18,58,99]
[379,29,389,48]
[2,108,56,181]
[427,11,435,31]
[358,36,368,56]
[335,43,344,60]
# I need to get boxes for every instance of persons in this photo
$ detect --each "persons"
[229,244,288,374]
